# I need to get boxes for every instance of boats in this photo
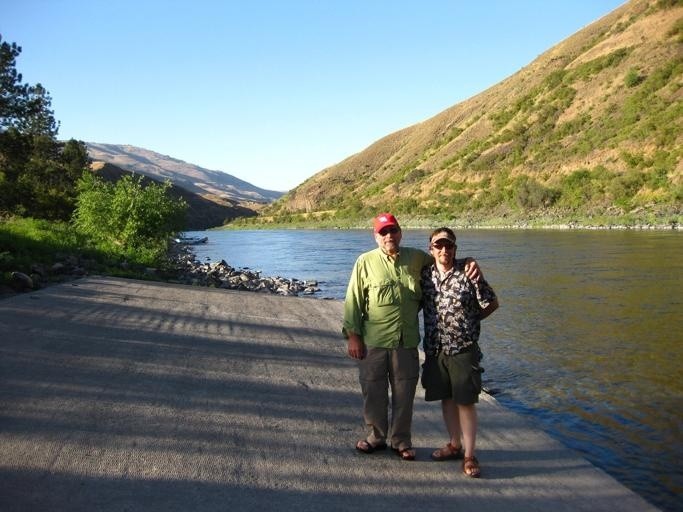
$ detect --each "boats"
[175,236,208,244]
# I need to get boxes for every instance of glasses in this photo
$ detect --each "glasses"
[431,244,454,250]
[379,227,399,236]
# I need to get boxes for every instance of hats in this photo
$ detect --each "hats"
[429,230,455,246]
[373,213,398,233]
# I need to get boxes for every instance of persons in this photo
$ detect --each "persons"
[417,227,498,479]
[338,211,482,461]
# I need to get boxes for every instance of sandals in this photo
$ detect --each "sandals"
[390,442,416,460]
[356,438,387,453]
[431,444,464,461]
[463,451,481,478]
